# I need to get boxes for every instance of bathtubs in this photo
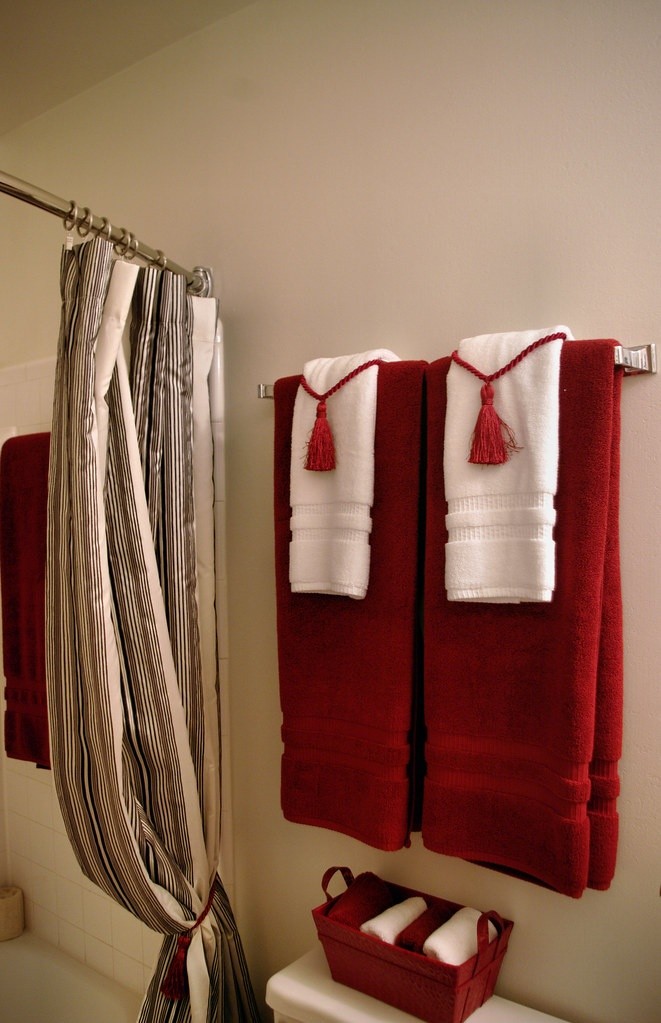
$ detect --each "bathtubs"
[1,932,143,1023]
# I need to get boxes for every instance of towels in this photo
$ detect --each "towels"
[325,871,496,966]
[273,325,623,898]
[0,431,51,769]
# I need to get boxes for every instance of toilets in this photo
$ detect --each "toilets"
[266,937,571,1023]
